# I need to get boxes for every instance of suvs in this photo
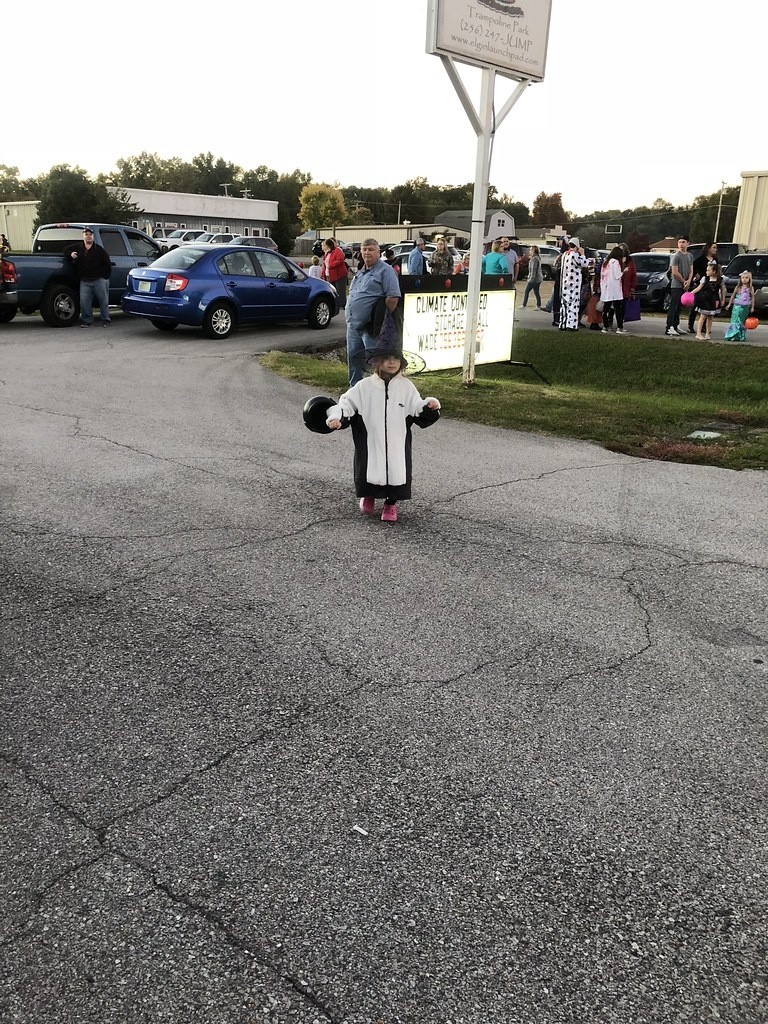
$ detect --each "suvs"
[311,238,345,258]
[149,226,278,259]
[515,244,562,281]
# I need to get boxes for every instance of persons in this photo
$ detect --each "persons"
[600,247,631,335]
[381,249,401,276]
[687,241,720,334]
[454,254,470,274]
[725,271,755,342]
[608,243,637,332]
[428,238,454,274]
[326,336,440,522]
[308,255,322,279]
[688,263,727,340]
[481,241,509,274]
[499,237,519,322]
[665,235,694,336]
[589,252,608,331]
[517,244,543,310]
[414,237,428,274]
[408,238,423,287]
[320,237,347,310]
[0,233,9,253]
[540,236,593,332]
[66,228,113,326]
[343,237,400,389]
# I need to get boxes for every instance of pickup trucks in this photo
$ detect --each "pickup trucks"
[0,220,169,327]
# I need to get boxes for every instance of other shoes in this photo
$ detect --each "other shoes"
[518,305,524,309]
[589,323,603,330]
[103,321,110,327]
[540,308,551,313]
[701,329,711,334]
[687,327,696,333]
[81,323,94,327]
[359,498,375,512]
[381,503,397,520]
[695,335,706,340]
[533,307,540,311]
[705,335,711,339]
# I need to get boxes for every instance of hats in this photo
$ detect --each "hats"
[367,307,408,369]
[83,227,94,233]
[417,238,424,244]
[568,238,579,248]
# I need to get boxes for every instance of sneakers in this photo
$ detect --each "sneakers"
[676,328,687,335]
[665,326,680,336]
[616,328,630,334]
[601,327,616,333]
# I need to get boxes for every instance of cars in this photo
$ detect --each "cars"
[119,244,341,340]
[339,242,362,259]
[595,242,768,322]
[353,240,468,276]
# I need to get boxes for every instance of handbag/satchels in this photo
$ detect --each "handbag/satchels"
[623,296,641,322]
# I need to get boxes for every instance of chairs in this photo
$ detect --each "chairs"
[229,255,246,274]
